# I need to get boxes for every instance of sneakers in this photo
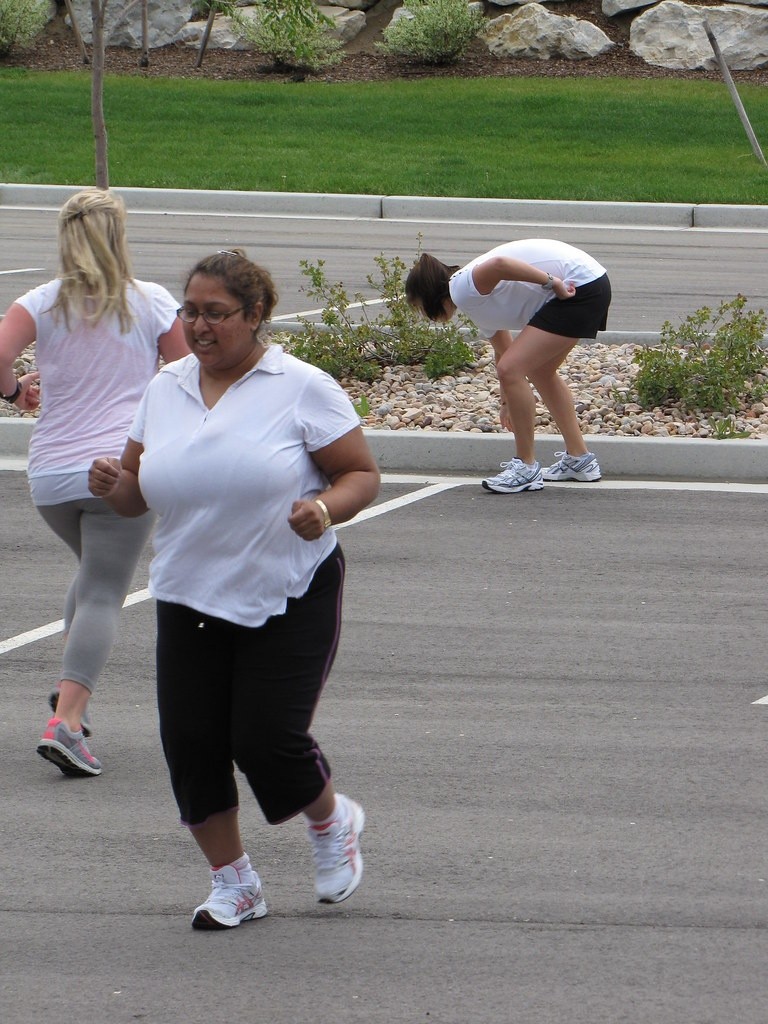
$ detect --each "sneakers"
[484,457,543,492]
[541,450,602,481]
[309,793,365,902]
[37,717,100,776]
[191,863,269,930]
[49,688,93,738]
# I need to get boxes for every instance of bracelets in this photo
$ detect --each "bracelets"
[316,500,332,527]
[0,381,22,403]
[502,402,506,405]
[542,273,553,290]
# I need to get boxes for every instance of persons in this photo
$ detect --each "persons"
[406,239,612,493]
[0,190,190,773]
[87,250,381,931]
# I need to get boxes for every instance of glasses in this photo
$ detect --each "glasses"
[177,304,248,324]
